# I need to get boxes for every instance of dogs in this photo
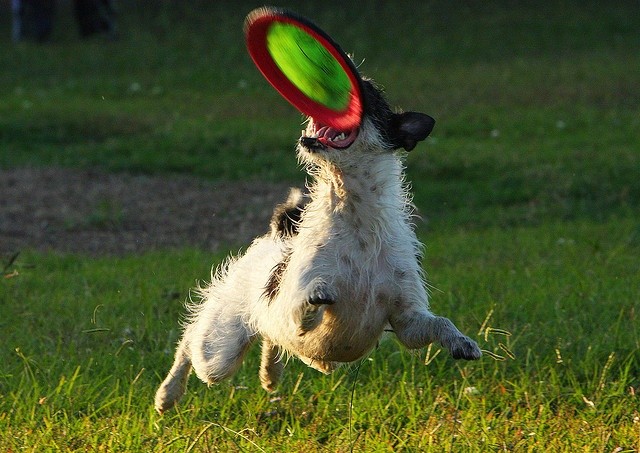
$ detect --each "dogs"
[154,52,484,416]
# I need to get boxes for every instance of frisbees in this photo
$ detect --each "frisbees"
[243,5,366,131]
